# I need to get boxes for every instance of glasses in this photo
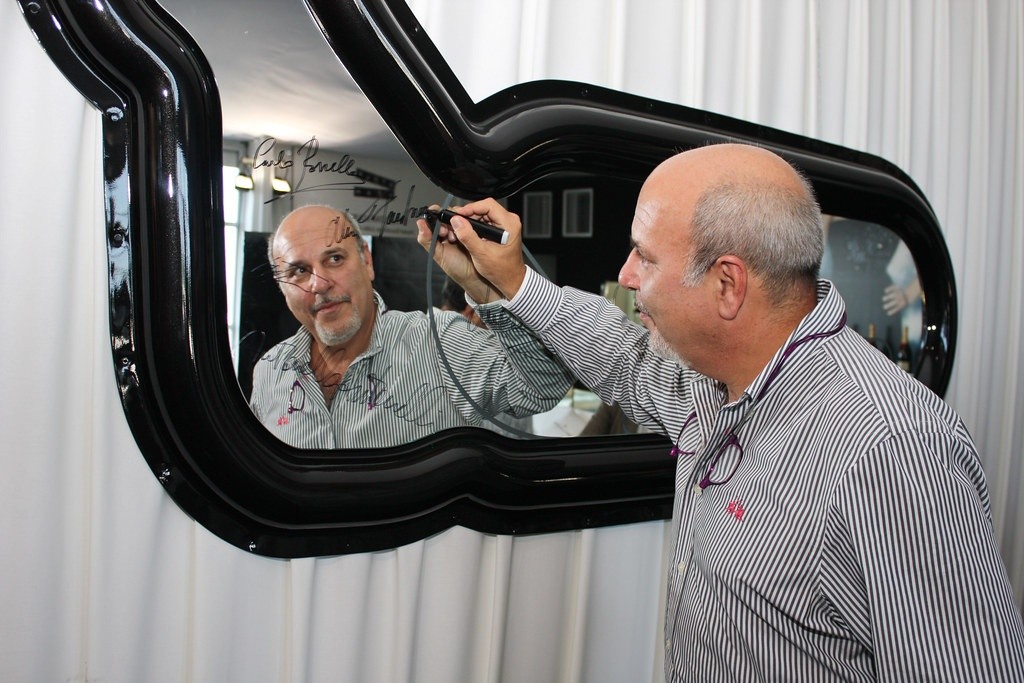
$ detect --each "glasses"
[286,356,377,413]
[671,410,744,489]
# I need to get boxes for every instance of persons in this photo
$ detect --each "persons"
[250,204,576,450]
[817,215,923,374]
[451,142,1024,683]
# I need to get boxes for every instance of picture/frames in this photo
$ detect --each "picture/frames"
[521,191,551,240]
[560,187,594,237]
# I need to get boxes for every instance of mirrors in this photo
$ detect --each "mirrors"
[18,0,958,559]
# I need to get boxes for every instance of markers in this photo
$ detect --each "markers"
[426,207,509,246]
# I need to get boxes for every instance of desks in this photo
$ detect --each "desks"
[532,389,612,437]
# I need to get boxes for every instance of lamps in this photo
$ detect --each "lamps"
[234,156,255,191]
[271,160,291,194]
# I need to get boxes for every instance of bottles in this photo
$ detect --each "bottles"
[881,326,894,362]
[897,328,911,372]
[865,324,877,349]
